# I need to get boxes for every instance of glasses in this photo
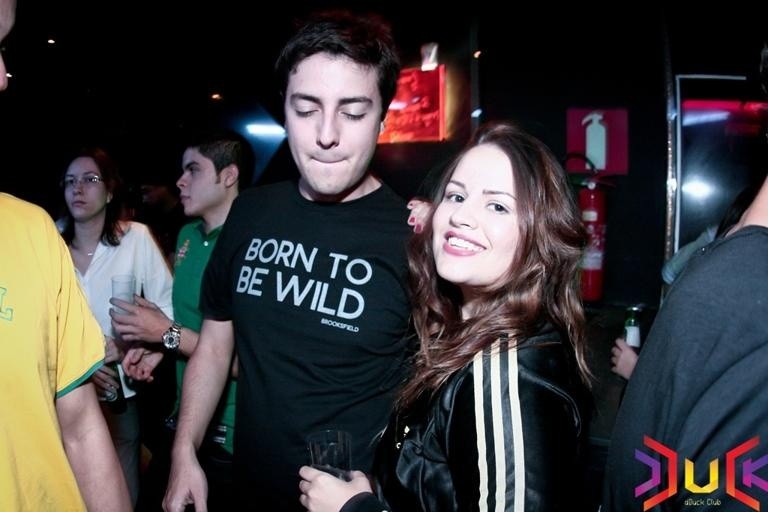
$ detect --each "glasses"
[60,175,104,192]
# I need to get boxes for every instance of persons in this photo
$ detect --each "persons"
[0,1,767,512]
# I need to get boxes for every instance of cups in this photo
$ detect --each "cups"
[307,428,353,487]
[111,275,136,315]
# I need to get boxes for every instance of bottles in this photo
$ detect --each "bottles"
[623,307,643,356]
[104,362,128,414]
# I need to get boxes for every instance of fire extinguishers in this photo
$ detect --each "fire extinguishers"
[561,152,615,301]
